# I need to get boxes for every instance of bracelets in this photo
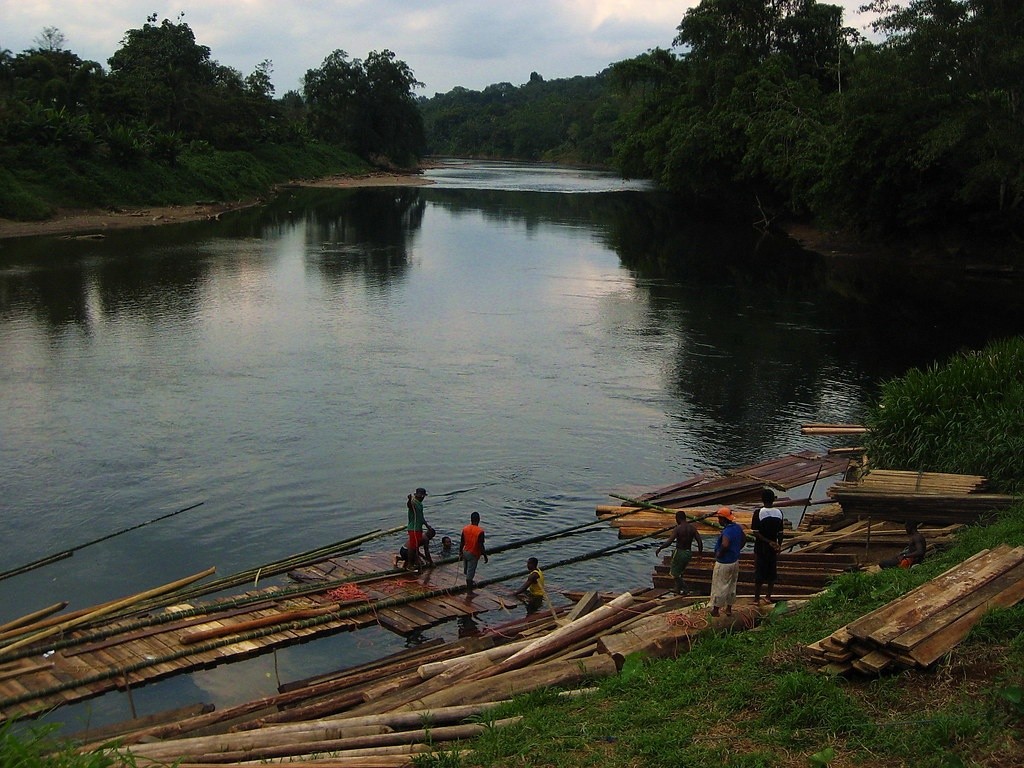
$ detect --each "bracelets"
[904,554,905,557]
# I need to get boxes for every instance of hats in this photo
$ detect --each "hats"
[718,507,734,521]
[414,487,428,497]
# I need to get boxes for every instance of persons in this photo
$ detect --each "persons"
[394,528,436,568]
[709,507,747,617]
[878,520,926,570]
[746,488,784,605]
[656,511,703,594]
[515,557,544,596]
[442,536,452,548]
[459,512,488,597]
[407,488,432,572]
